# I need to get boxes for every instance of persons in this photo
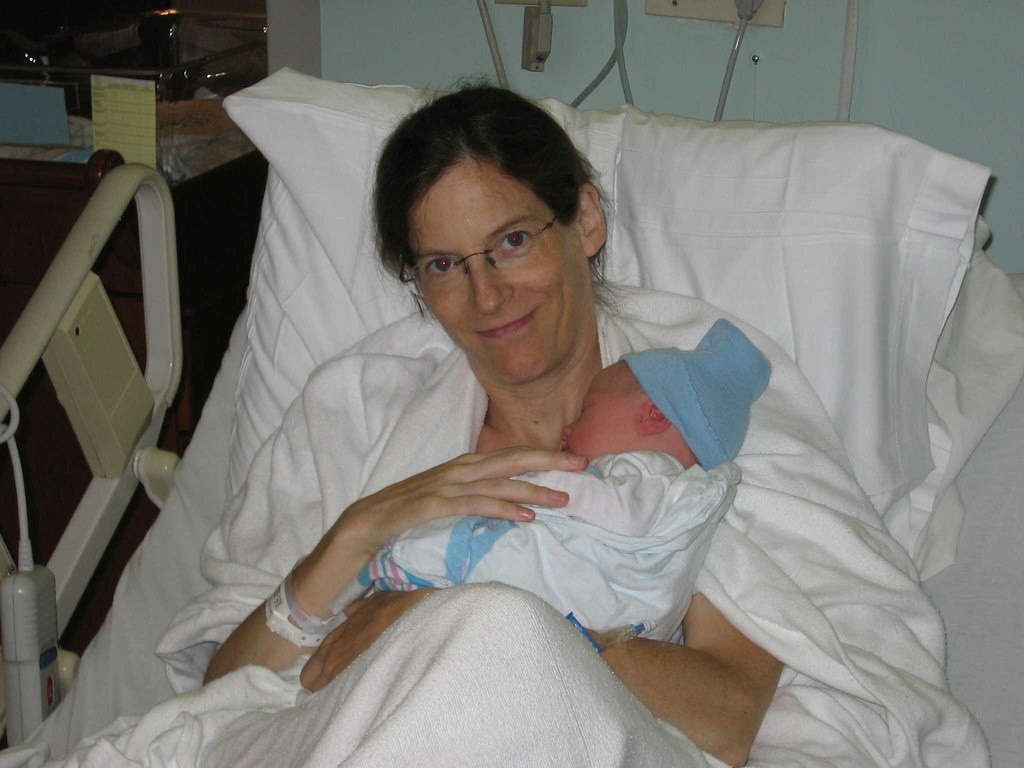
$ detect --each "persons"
[150,85,786,768]
[355,317,772,643]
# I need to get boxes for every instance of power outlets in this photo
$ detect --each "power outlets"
[644,0,787,29]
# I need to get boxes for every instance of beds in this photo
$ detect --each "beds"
[0,162,1024,768]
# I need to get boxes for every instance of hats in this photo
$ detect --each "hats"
[619,319,773,472]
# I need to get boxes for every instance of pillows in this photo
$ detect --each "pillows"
[222,67,994,518]
[913,213,1024,582]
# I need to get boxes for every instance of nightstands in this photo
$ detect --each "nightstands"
[0,97,268,655]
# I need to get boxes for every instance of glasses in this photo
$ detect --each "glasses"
[399,215,557,299]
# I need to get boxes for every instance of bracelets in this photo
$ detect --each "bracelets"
[262,569,335,647]
